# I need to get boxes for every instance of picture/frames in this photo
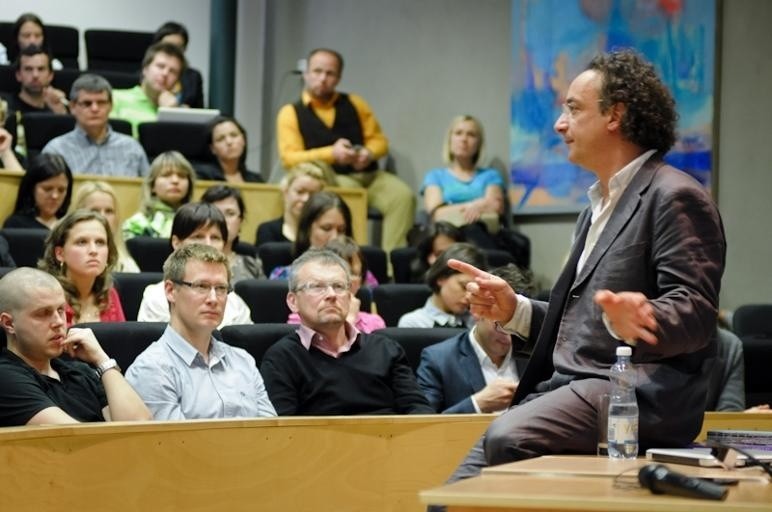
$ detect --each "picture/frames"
[510,0,724,217]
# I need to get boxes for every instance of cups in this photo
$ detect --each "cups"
[598,395,610,458]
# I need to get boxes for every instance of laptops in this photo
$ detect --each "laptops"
[157,108,222,124]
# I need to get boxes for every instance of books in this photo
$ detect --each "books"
[645,446,772,467]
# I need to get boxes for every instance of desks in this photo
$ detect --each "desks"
[419,450,772,512]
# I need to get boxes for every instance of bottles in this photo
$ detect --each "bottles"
[608,345,639,457]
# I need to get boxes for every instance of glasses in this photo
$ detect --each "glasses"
[562,98,606,116]
[171,278,232,295]
[74,100,111,110]
[293,280,350,294]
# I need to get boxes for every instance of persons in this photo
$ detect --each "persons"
[0,152,435,425]
[0,12,70,169]
[705,310,745,413]
[42,74,151,176]
[401,115,532,414]
[190,115,265,181]
[105,23,204,141]
[278,49,417,281]
[426,46,726,512]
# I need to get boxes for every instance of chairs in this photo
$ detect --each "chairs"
[0,23,213,170]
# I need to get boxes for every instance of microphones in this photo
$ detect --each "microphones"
[639,463,728,500]
